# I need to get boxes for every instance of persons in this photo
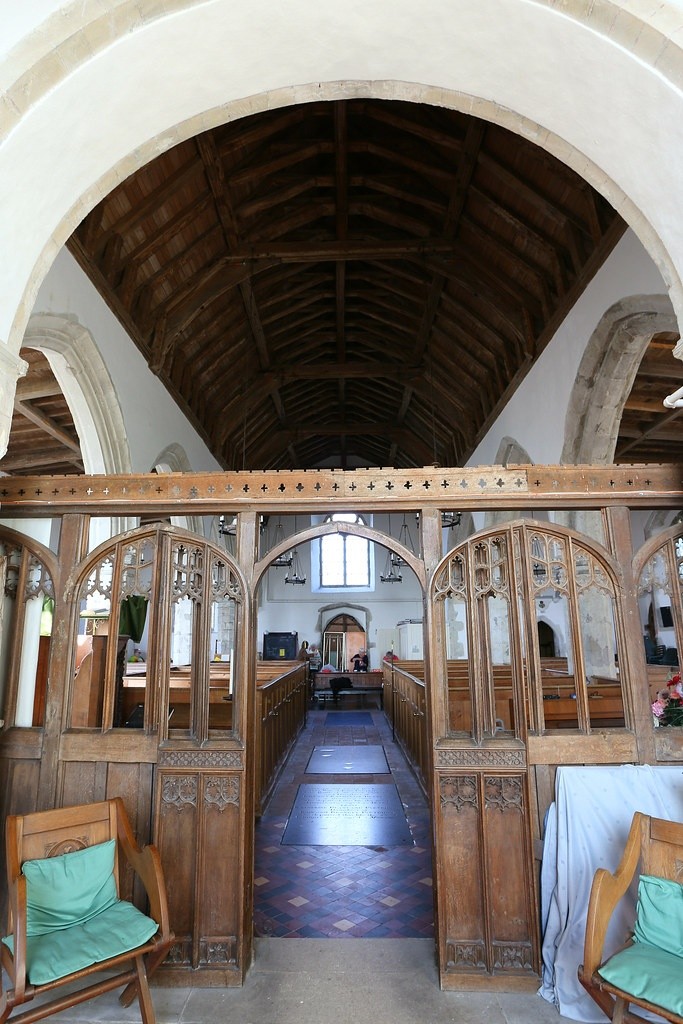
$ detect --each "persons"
[643,631,656,657]
[350,648,369,671]
[297,641,318,662]
[383,651,399,661]
[128,649,145,662]
[307,643,320,657]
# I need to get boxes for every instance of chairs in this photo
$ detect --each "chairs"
[0,797,176,1024]
[578,812,683,1024]
[644,636,678,666]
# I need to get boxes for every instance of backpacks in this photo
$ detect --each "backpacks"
[329,677,353,700]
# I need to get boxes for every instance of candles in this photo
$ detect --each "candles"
[141,553,143,560]
[229,649,234,694]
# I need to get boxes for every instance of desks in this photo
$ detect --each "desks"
[313,672,383,689]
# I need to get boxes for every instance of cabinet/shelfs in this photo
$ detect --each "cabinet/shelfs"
[263,632,299,659]
[31,635,129,727]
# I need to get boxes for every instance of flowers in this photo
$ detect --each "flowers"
[651,673,683,727]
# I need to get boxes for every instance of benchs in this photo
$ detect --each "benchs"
[118,659,310,821]
[383,657,683,807]
[314,689,383,710]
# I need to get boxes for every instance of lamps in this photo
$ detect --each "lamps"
[380,514,403,584]
[270,515,293,569]
[415,278,462,530]
[530,510,546,577]
[218,278,264,538]
[389,513,420,568]
[284,515,307,586]
